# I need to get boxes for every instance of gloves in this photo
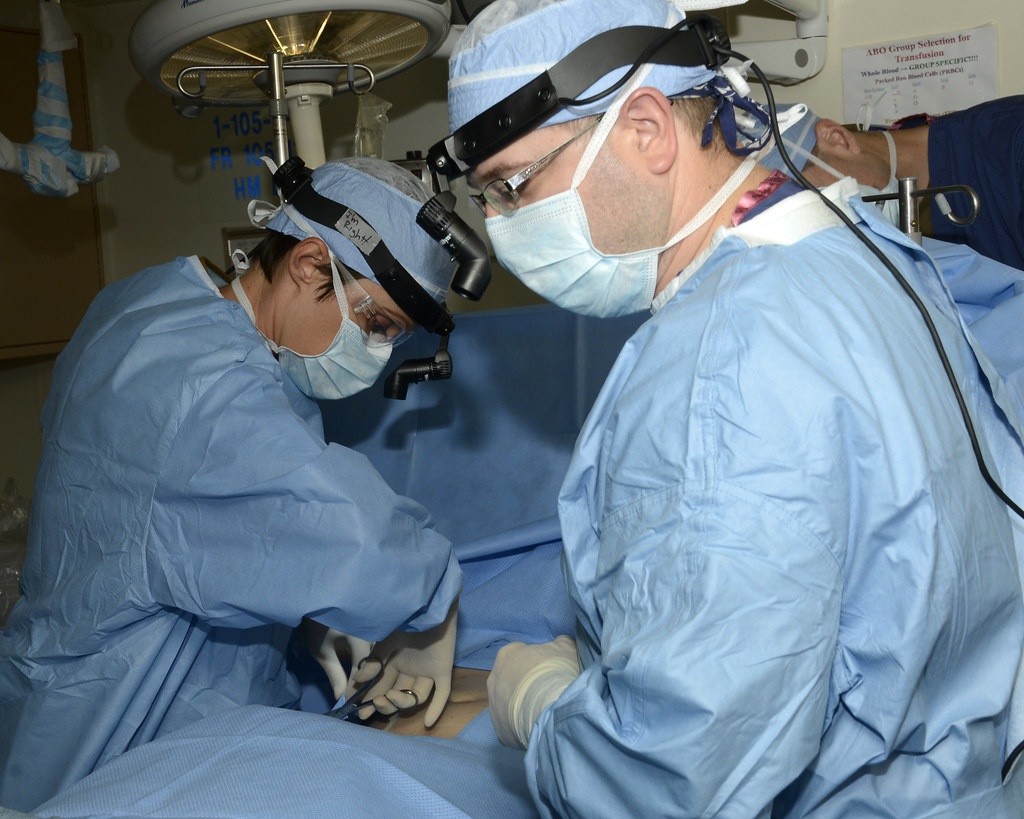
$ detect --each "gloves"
[288,617,376,700]
[353,595,459,728]
[485,634,580,751]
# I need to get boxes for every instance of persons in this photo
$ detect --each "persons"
[1,94,1023,819]
[1,159,466,811]
[450,1,1024,819]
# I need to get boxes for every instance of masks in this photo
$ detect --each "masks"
[243,262,393,400]
[484,108,696,319]
[814,163,902,230]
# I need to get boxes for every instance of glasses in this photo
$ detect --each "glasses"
[469,97,674,220]
[332,256,414,349]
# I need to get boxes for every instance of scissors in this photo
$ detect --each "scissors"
[322,655,419,721]
